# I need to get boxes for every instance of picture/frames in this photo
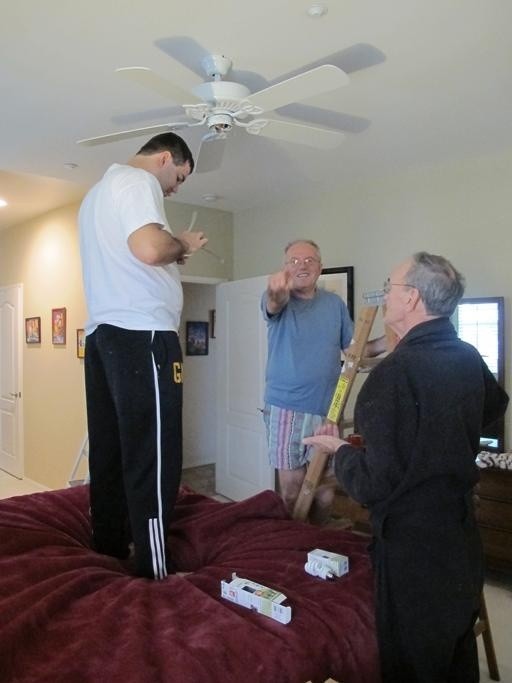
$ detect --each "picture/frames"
[26,317,41,344]
[52,308,66,345]
[77,329,85,357]
[186,321,208,355]
[316,266,354,322]
[212,309,216,337]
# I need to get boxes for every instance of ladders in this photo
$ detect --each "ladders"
[292,287,501,681]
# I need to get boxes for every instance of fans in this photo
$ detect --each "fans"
[76,53,349,150]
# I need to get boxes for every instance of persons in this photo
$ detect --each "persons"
[301,251,510,683]
[78,132,208,583]
[260,239,387,527]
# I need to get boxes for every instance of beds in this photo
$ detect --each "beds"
[0,482,383,683]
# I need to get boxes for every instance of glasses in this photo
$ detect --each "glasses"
[383,278,424,292]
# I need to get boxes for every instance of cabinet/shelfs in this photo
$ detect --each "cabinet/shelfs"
[478,467,512,583]
